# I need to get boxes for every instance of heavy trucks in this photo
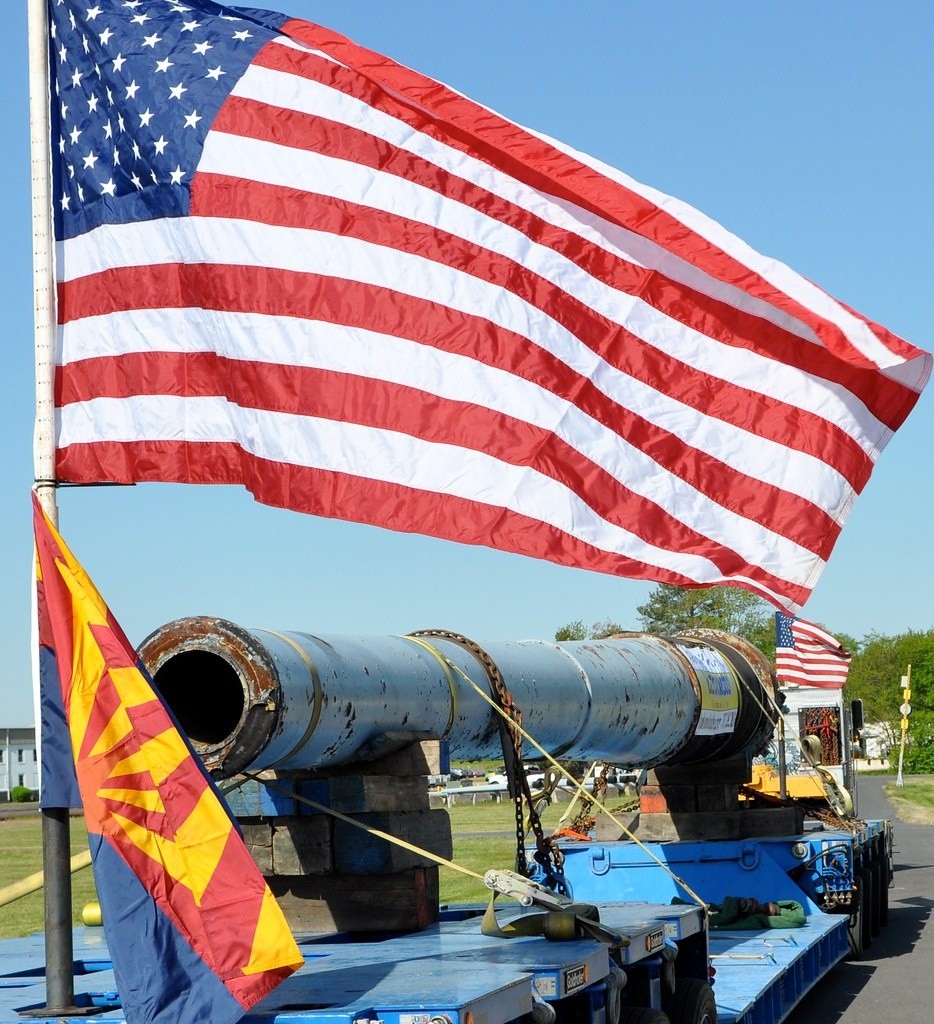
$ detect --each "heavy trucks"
[1,685,898,1024]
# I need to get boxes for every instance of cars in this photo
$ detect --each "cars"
[452,765,544,789]
[607,775,637,792]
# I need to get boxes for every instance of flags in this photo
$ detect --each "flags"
[48,1,934,615]
[30,490,306,1024]
[776,611,852,691]
